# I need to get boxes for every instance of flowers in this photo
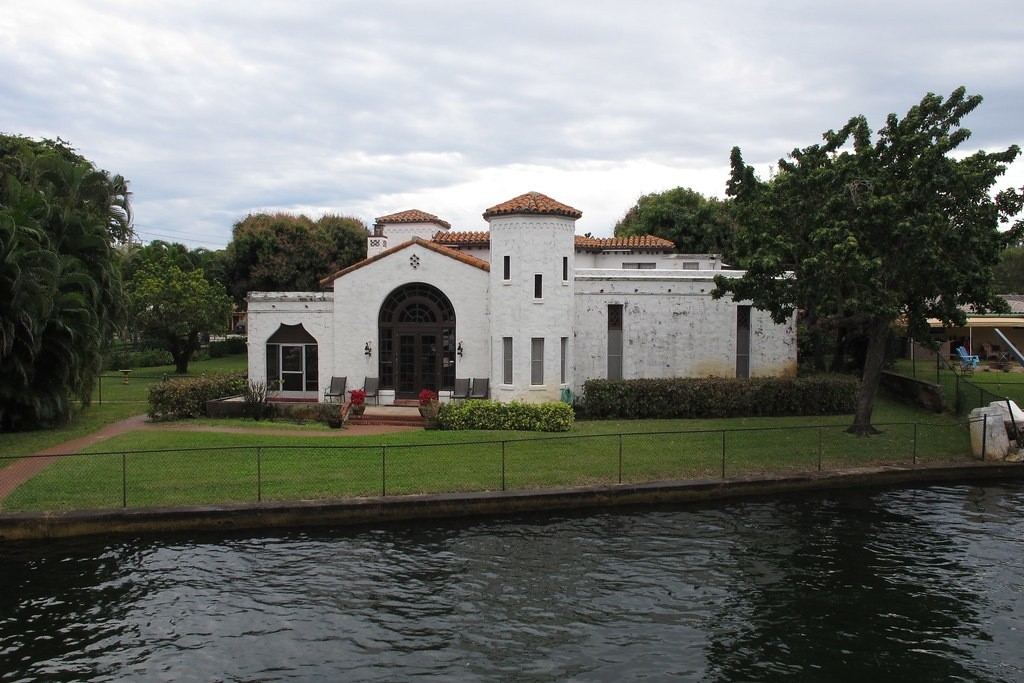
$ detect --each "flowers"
[418,390,439,407]
[351,389,366,407]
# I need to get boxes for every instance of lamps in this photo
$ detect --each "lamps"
[365,344,372,356]
[456,343,463,356]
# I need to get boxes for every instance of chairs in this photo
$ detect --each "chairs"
[467,378,489,399]
[358,377,380,407]
[960,346,979,365]
[323,376,347,403]
[956,348,977,369]
[449,378,470,400]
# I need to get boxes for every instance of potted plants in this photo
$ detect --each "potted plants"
[420,405,441,430]
[322,401,345,428]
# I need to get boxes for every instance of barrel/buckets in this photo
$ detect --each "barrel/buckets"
[969,406,1010,460]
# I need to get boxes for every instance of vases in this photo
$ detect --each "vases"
[418,404,441,416]
[352,406,365,415]
